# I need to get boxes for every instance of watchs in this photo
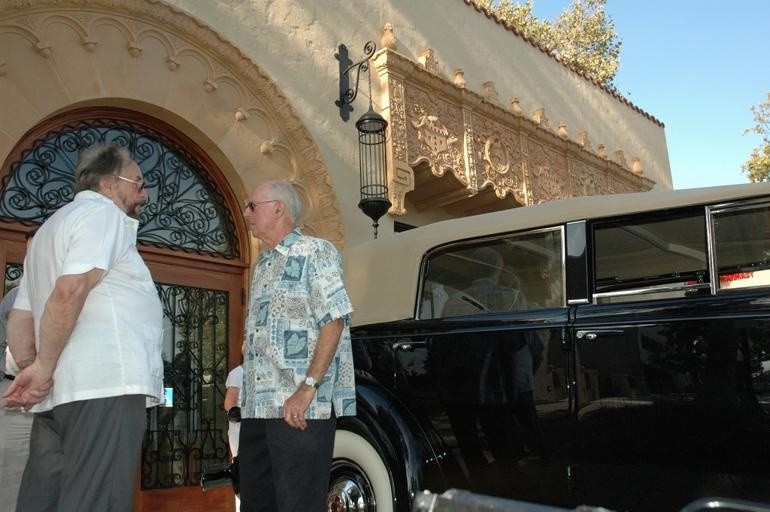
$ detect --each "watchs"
[300,376,320,391]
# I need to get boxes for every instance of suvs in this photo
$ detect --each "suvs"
[226,180,768,510]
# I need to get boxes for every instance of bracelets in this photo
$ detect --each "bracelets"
[15,356,36,370]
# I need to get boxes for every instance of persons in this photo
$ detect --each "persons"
[0,228,38,512]
[439,248,529,316]
[232,178,360,512]
[225,338,247,512]
[1,144,166,512]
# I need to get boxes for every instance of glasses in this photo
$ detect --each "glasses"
[118,172,146,193]
[246,200,277,212]
